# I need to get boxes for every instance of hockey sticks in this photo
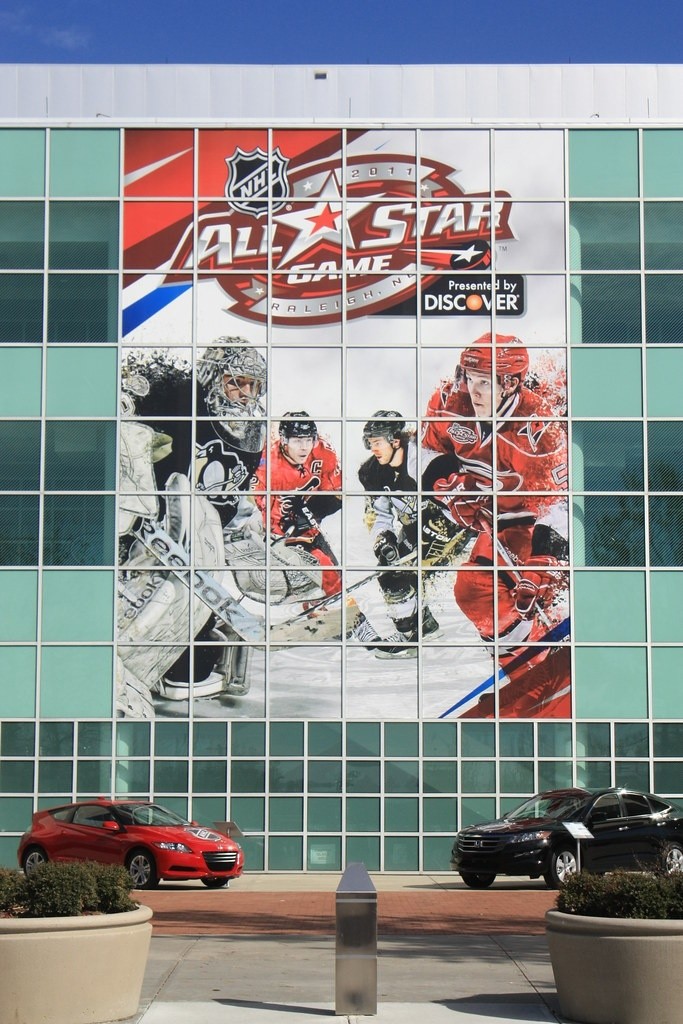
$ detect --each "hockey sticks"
[235,524,295,606]
[128,514,361,652]
[281,550,419,625]
[477,512,555,631]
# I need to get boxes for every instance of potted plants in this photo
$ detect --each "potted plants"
[0,860,153,1024]
[543,840,682,1024]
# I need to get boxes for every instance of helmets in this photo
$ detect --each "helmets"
[278,412,319,444]
[460,334,529,374]
[362,410,404,451]
[198,337,268,398]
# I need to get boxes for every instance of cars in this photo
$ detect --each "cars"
[450,787,683,890]
[17,800,244,890]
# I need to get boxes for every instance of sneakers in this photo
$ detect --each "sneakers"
[353,613,381,651]
[478,667,572,721]
[374,607,443,659]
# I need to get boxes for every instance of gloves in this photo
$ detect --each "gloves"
[516,555,568,616]
[278,492,343,538]
[432,472,490,528]
[372,532,399,564]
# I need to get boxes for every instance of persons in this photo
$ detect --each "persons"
[359,409,471,661]
[412,332,570,718]
[241,411,382,649]
[119,337,272,698]
